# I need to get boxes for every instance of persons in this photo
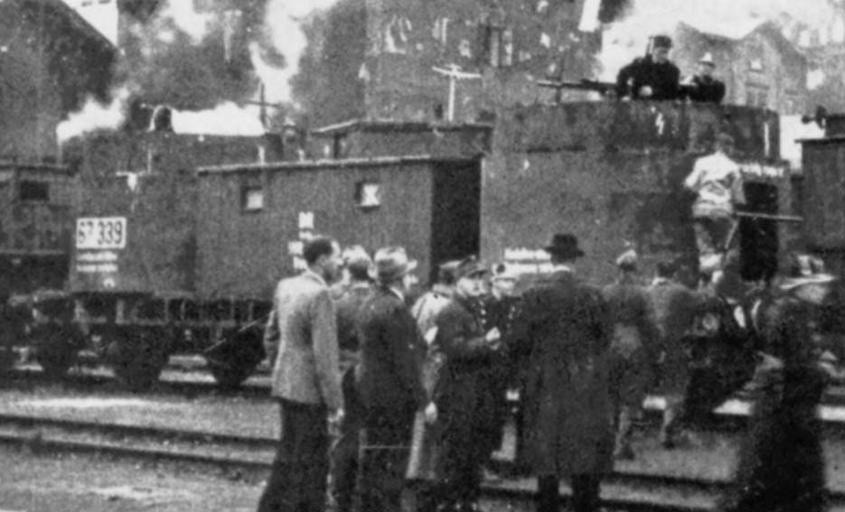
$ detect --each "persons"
[405,254,531,512]
[251,234,343,512]
[324,244,437,512]
[683,130,747,266]
[714,311,833,512]
[599,247,660,461]
[509,232,623,512]
[612,35,680,100]
[679,50,726,105]
[646,256,728,450]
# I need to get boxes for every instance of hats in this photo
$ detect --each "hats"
[656,257,679,272]
[544,233,584,256]
[454,255,487,280]
[617,249,638,269]
[366,245,419,282]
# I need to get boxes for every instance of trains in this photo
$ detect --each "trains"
[1,70,842,413]
